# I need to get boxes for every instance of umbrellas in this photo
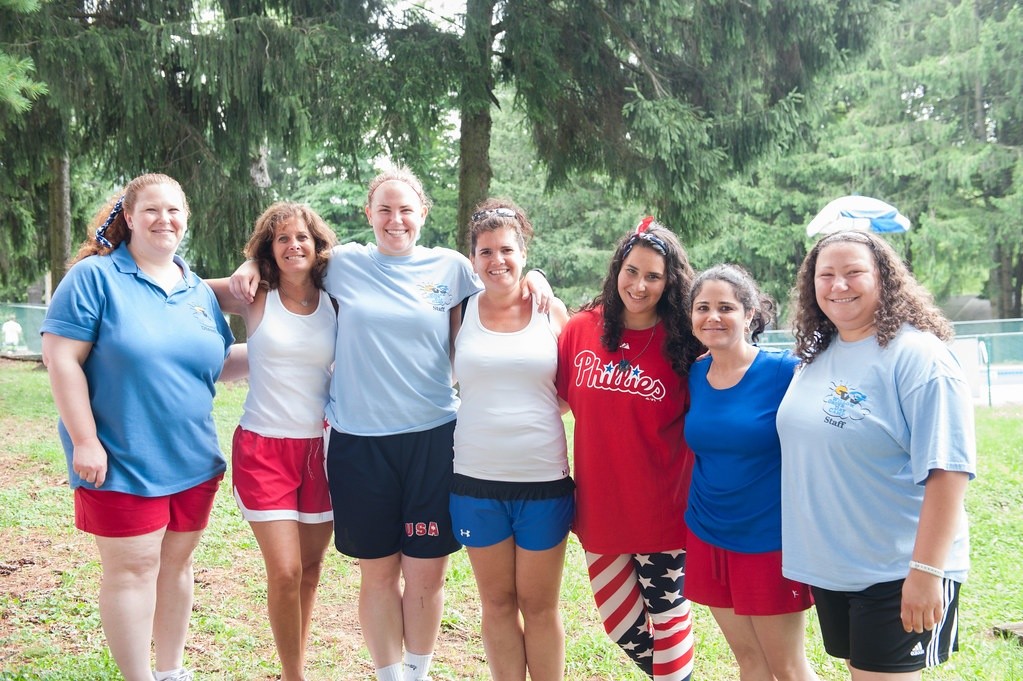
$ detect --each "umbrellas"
[805,195,911,238]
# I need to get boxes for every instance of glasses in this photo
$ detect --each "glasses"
[472,207,517,223]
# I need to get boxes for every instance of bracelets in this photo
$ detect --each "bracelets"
[534,269,547,278]
[909,560,944,578]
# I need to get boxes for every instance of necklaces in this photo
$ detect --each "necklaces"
[620,313,657,371]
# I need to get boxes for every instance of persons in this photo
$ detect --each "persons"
[201,198,340,681]
[229,171,553,681]
[1,314,24,345]
[450,197,570,681]
[554,218,707,681]
[40,173,248,681]
[695,229,975,681]
[684,264,816,681]
